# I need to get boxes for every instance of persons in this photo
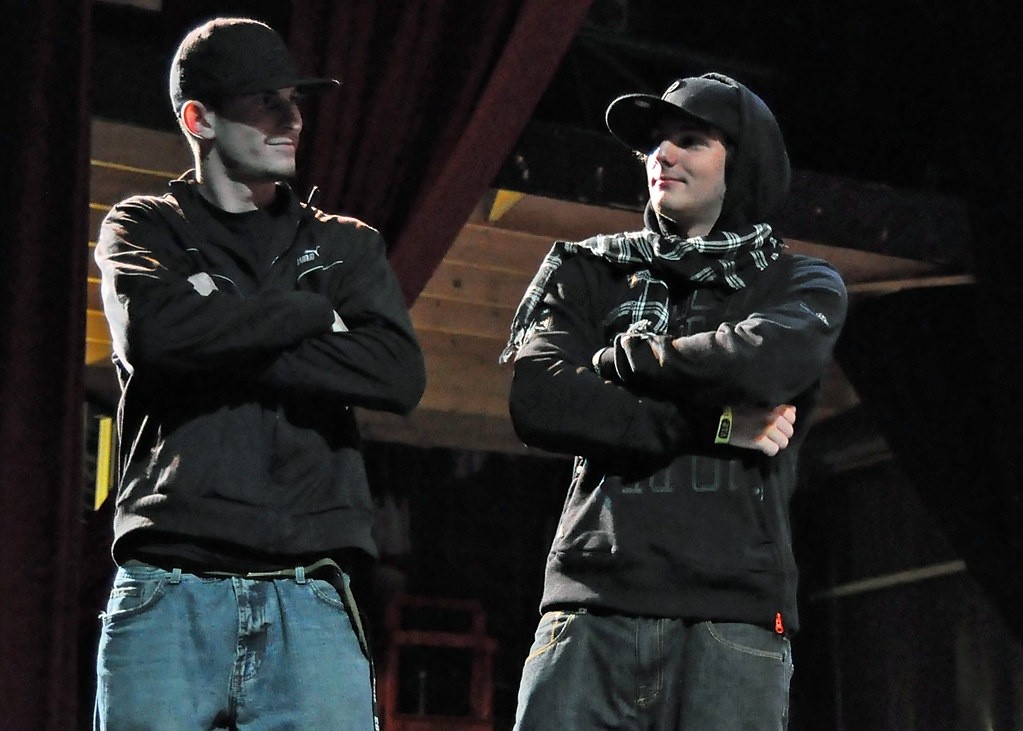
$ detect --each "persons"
[89,12,430,730]
[495,68,848,731]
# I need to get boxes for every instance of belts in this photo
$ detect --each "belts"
[194,558,369,661]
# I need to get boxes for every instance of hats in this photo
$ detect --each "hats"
[605,72,745,146]
[167,18,340,107]
